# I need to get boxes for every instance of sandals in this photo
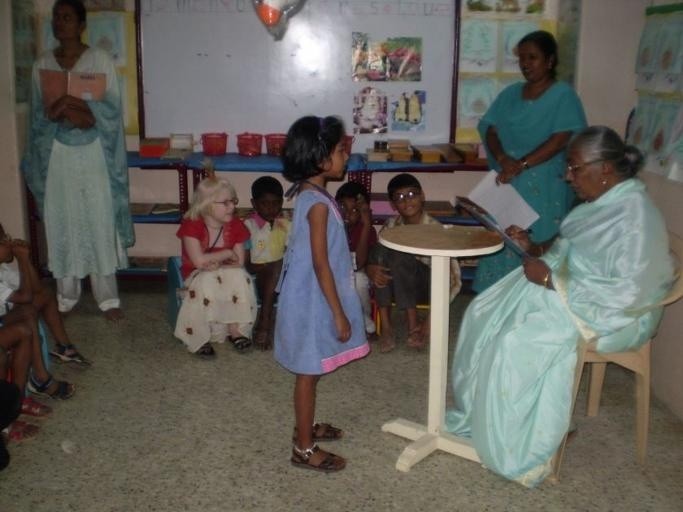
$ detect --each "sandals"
[292,423,343,442]
[291,444,346,472]
[407,324,423,347]
[192,334,253,356]
[1,340,89,470]
[381,333,395,353]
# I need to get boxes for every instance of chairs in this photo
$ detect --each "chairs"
[371,298,376,320]
[376,297,430,336]
[546,227,683,484]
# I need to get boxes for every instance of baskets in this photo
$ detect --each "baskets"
[345,135,354,154]
[238,132,261,156]
[265,133,286,156]
[201,132,228,155]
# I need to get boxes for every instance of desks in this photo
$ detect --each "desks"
[377,224,505,473]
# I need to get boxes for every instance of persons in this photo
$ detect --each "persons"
[470,31,588,293]
[175,176,259,359]
[444,126,674,490]
[335,181,379,334]
[244,176,290,350]
[0,226,92,470]
[271,115,372,472]
[20,0,136,321]
[364,175,464,353]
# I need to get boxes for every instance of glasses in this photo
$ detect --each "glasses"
[568,159,600,173]
[212,198,239,206]
[395,191,422,202]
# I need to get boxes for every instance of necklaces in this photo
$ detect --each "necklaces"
[204,223,223,230]
[526,82,548,105]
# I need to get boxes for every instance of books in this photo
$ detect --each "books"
[454,167,540,261]
[39,69,107,106]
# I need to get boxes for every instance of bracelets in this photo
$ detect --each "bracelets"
[544,271,550,289]
[520,157,529,169]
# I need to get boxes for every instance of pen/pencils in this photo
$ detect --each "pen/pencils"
[508,228,534,236]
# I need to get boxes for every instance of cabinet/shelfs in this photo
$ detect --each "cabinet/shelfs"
[185,153,365,226]
[82,150,190,297]
[362,155,489,280]
[18,148,185,282]
